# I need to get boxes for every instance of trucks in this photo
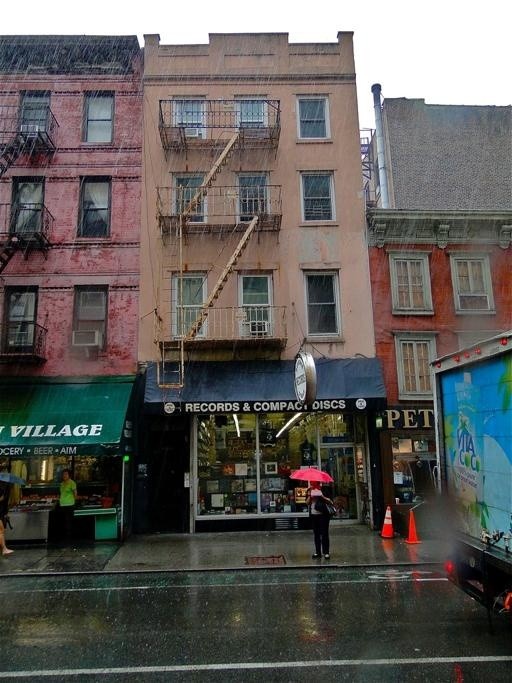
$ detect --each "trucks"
[425,328,512,624]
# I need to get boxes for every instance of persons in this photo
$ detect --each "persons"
[0,481,15,556]
[58,468,81,549]
[305,480,334,559]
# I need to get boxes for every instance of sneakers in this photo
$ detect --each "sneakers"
[2,548,14,556]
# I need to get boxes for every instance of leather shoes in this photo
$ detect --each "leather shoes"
[324,553,330,558]
[312,553,320,557]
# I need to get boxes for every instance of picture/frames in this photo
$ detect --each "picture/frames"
[264,460,279,475]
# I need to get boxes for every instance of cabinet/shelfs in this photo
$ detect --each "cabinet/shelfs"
[4,495,130,543]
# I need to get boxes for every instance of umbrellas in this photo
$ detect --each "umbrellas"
[0,471,29,486]
[289,467,336,483]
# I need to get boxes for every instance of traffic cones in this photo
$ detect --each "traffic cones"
[449,660,465,682]
[405,543,418,564]
[378,504,397,539]
[402,508,423,543]
[381,537,396,561]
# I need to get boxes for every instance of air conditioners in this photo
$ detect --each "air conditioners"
[71,329,103,350]
